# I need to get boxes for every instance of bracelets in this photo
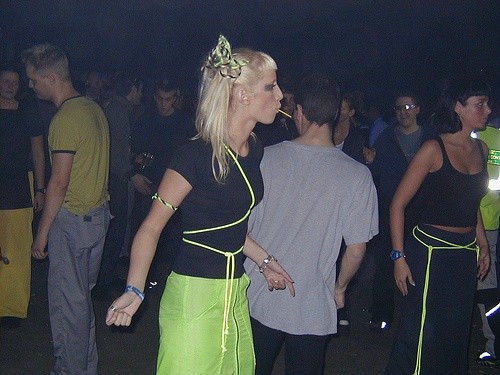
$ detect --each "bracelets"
[125,285,145,302]
[259,254,277,273]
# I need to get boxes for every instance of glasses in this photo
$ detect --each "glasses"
[392,104,417,112]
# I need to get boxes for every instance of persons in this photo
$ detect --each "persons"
[385,76,492,375]
[0,62,46,329]
[23,42,111,375]
[251,79,294,147]
[332,87,500,365]
[243,73,379,375]
[84,64,196,303]
[106,34,295,375]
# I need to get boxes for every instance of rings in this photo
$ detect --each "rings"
[111,306,117,312]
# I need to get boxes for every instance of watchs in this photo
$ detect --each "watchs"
[35,187,46,194]
[390,250,406,261]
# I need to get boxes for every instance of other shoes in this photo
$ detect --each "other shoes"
[368,314,392,333]
[0,316,23,330]
[476,354,497,368]
